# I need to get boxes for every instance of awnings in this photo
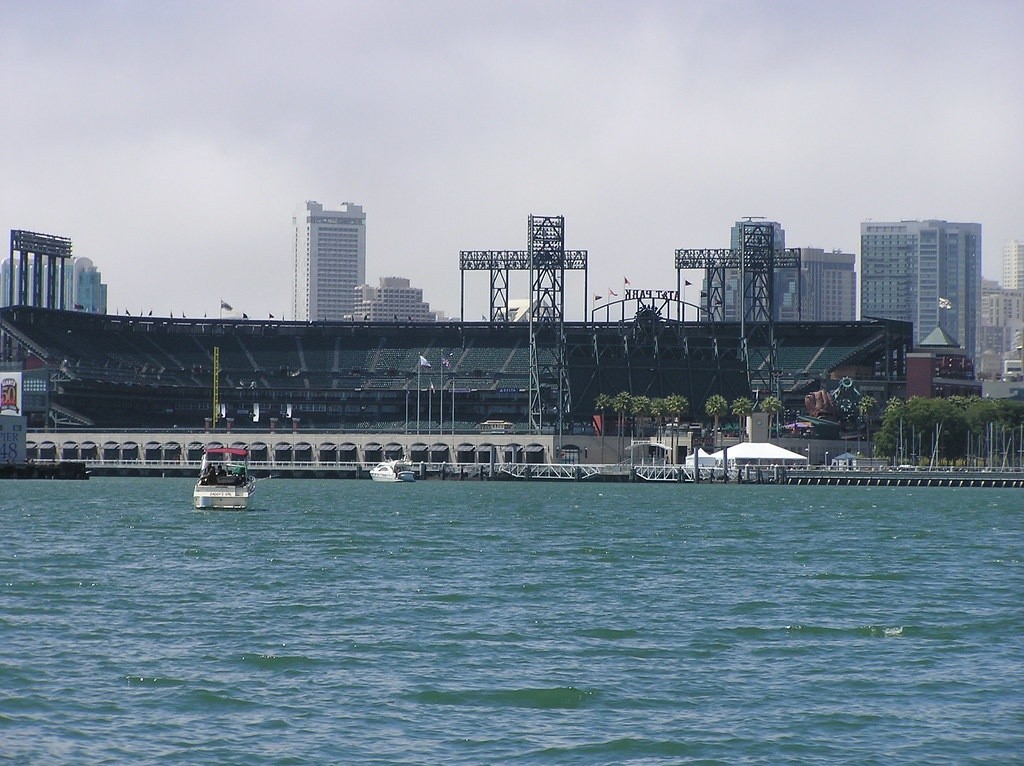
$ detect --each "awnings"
[141,444,266,450]
[454,445,543,452]
[273,444,355,451]
[26,444,136,450]
[360,445,401,452]
[408,445,449,451]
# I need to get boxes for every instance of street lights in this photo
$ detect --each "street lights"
[824,451,829,470]
[804,443,811,469]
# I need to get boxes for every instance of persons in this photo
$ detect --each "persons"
[202,464,227,484]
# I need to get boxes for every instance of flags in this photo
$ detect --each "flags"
[939,297,952,309]
[441,354,451,368]
[93,306,102,312]
[609,290,617,296]
[221,301,235,311]
[624,279,630,284]
[595,295,602,300]
[420,356,431,367]
[75,304,85,309]
[685,281,691,286]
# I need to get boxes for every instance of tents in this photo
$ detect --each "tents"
[709,442,808,468]
[836,453,858,470]
[685,448,716,467]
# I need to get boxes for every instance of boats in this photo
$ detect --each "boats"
[370,459,414,482]
[192,447,256,512]
